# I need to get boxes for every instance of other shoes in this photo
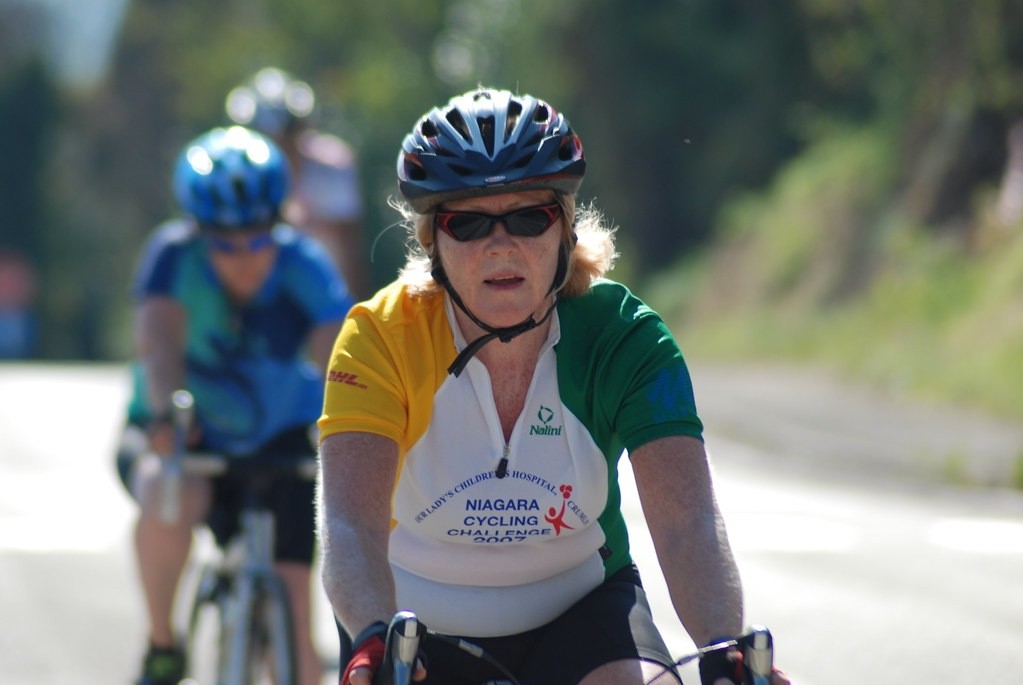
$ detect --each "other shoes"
[136,644,184,685]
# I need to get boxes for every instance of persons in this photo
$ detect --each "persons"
[0,253,36,354]
[114,129,354,685]
[224,65,366,373]
[319,84,790,684]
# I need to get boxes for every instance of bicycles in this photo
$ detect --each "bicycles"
[156,385,335,685]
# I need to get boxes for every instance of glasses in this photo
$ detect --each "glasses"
[435,204,562,241]
[212,234,273,253]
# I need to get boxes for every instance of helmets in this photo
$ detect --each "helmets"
[172,125,290,225]
[396,87,587,214]
